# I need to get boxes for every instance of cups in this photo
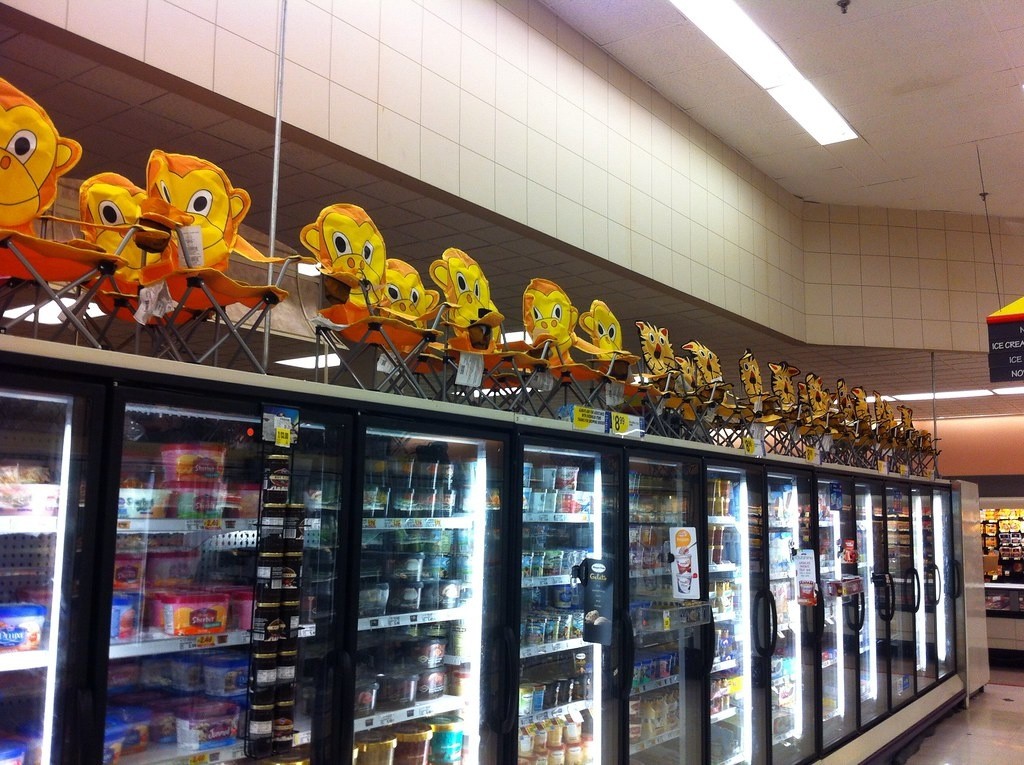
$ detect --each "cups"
[676,555,691,595]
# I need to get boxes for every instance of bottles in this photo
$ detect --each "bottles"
[519,714,593,765]
[710,678,730,715]
[641,689,679,737]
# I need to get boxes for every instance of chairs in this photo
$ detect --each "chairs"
[303,203,942,478]
[0,79,315,377]
